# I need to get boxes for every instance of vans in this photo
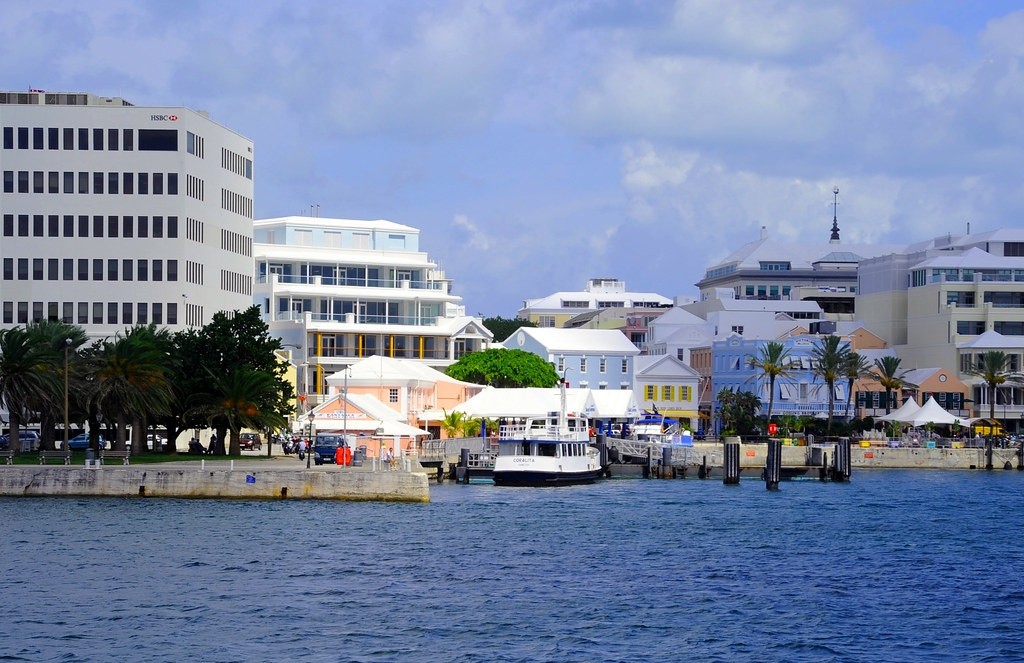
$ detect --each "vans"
[239,432,263,451]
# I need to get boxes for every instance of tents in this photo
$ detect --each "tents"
[871,395,971,427]
[957,417,1000,427]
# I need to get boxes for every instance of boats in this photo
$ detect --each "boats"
[491,378,615,484]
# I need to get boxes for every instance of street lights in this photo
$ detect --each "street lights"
[306,410,316,469]
[63,336,72,451]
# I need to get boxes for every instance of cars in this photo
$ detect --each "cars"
[0,430,42,449]
[125,433,168,453]
[59,433,107,451]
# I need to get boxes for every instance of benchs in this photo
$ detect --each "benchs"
[99,450,131,465]
[39,450,74,465]
[384,463,398,471]
[0,450,15,465]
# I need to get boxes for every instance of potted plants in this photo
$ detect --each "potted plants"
[924,421,936,448]
[949,419,961,448]
[794,416,815,446]
[778,412,795,446]
[883,420,901,449]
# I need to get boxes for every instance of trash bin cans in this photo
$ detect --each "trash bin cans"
[353,450,363,467]
[359,446,367,460]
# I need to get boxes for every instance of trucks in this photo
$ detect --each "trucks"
[315,432,352,465]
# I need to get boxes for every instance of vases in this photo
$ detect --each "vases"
[859,441,871,448]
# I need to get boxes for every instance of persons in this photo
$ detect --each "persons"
[384,447,396,468]
[295,439,306,452]
[589,426,597,437]
[187,434,217,456]
